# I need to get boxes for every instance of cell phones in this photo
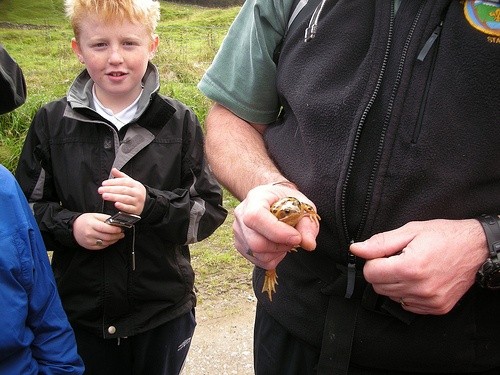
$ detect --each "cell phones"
[105,211,141,233]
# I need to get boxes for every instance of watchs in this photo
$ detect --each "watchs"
[476,213,500,291]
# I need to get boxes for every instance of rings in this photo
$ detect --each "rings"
[96,239,102,246]
[400,298,407,306]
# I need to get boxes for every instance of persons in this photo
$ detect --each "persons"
[0,164,85,375]
[197,0,500,375]
[15,0,229,375]
